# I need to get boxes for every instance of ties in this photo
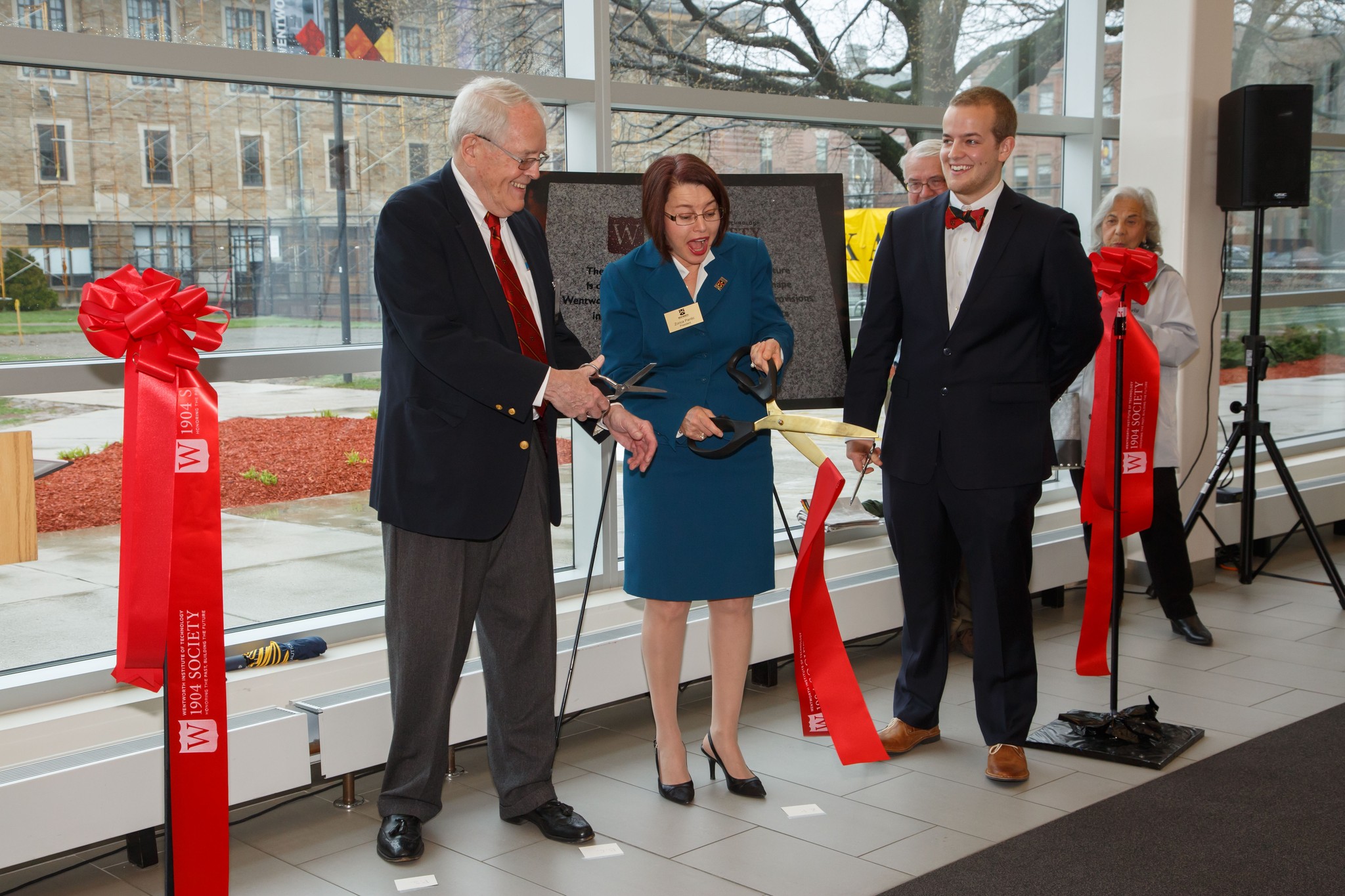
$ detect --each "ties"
[483,211,550,417]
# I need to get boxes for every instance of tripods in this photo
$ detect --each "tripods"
[1143,212,1344,611]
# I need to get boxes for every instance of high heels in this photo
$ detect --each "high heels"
[699,731,767,798]
[654,737,695,806]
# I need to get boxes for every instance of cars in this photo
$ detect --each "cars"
[1226,244,1345,270]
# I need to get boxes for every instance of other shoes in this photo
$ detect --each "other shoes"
[958,627,974,658]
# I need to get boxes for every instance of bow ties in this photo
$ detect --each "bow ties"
[945,208,987,232]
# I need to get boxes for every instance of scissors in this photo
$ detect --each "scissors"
[578,363,667,418]
[850,443,876,506]
[687,345,879,480]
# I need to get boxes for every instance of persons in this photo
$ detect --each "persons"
[600,156,794,804]
[899,139,979,665]
[1052,187,1215,650]
[369,77,658,864]
[841,88,1103,783]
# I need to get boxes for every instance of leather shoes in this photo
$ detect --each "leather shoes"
[1171,615,1213,646]
[504,798,595,842]
[985,744,1029,781]
[878,718,941,756]
[377,814,424,862]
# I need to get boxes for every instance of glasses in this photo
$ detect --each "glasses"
[665,208,721,226]
[904,176,946,194]
[474,134,550,171]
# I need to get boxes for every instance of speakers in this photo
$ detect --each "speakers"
[1213,82,1314,211]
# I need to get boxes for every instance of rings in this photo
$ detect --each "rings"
[750,362,756,368]
